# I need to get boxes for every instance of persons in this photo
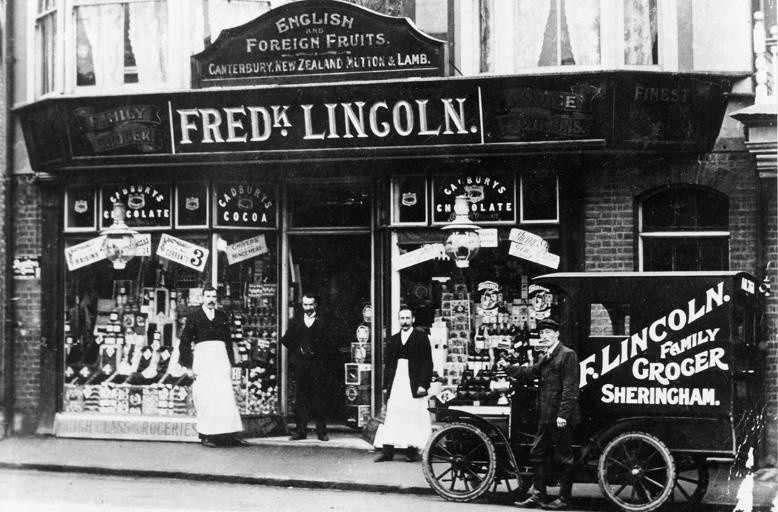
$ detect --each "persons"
[283,291,331,441]
[373,307,433,460]
[497,320,580,510]
[178,289,245,447]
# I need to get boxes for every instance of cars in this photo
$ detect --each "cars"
[423,271,767,510]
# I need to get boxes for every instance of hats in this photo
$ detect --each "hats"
[536,319,560,330]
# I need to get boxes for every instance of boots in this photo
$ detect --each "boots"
[514,476,547,507]
[540,482,572,510]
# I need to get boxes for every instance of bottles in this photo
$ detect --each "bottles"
[455,313,529,400]
[232,305,277,364]
[225,284,233,313]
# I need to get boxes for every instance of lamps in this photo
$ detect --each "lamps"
[99,202,139,271]
[440,195,482,268]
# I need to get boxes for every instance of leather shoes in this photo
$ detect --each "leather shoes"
[317,432,329,441]
[202,437,218,447]
[231,436,248,446]
[406,452,418,461]
[293,431,306,440]
[374,453,392,462]
